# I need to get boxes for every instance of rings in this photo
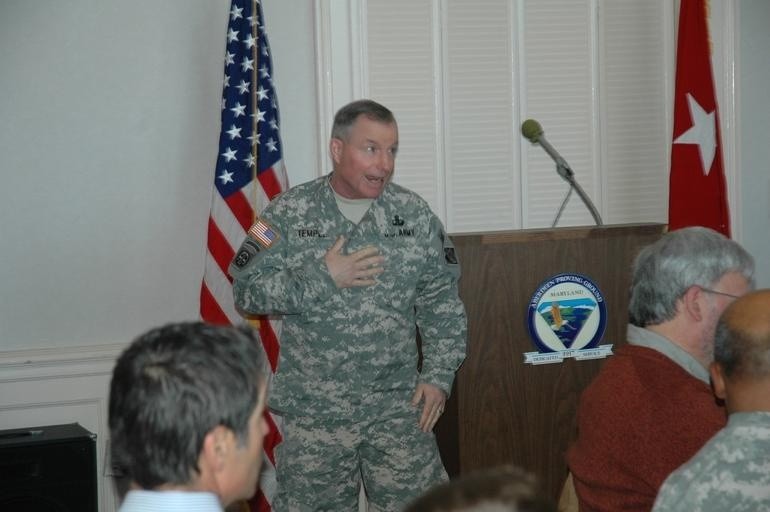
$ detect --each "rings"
[436,408,445,415]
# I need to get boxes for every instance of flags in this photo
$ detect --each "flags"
[667,2,735,240]
[197,1,290,511]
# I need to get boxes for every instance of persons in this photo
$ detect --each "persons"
[228,99,468,510]
[427,461,559,512]
[568,225,757,511]
[107,320,270,512]
[651,286,770,511]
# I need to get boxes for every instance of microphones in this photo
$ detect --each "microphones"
[522,120,573,176]
